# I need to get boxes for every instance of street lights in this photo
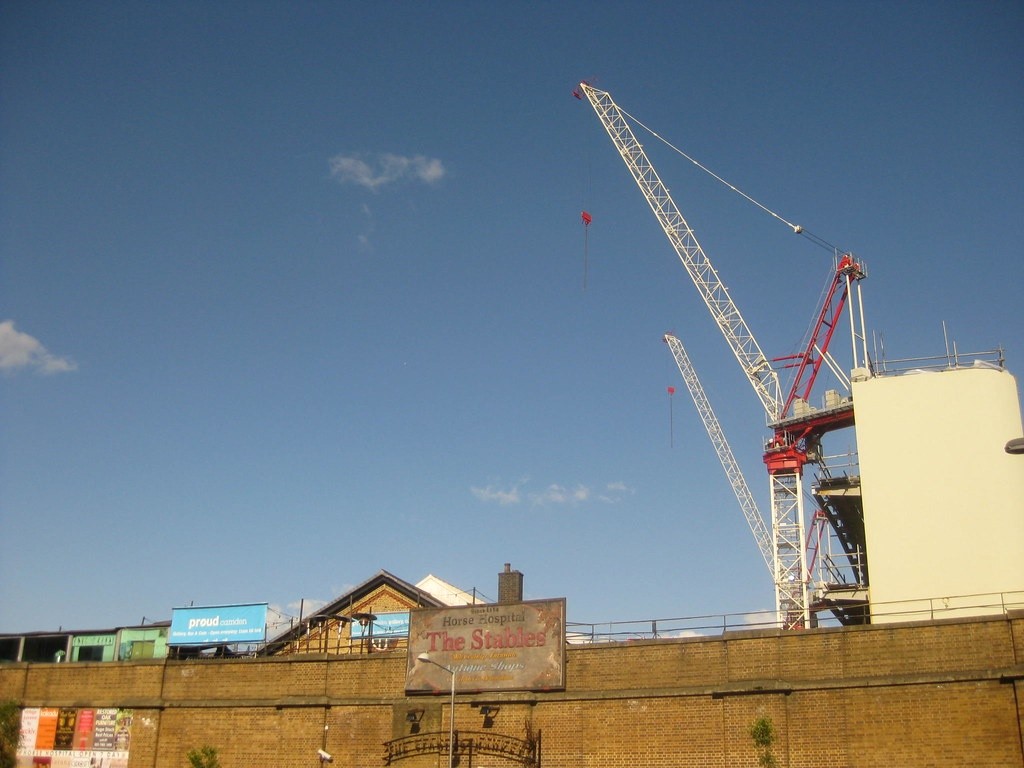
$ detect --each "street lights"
[417,649,455,768]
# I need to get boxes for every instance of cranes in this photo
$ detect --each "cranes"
[658,326,820,629]
[567,77,873,631]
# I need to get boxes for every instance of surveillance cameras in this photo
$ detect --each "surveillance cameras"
[318,749,332,761]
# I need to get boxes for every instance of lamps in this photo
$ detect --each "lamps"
[405,708,425,721]
[480,706,501,714]
